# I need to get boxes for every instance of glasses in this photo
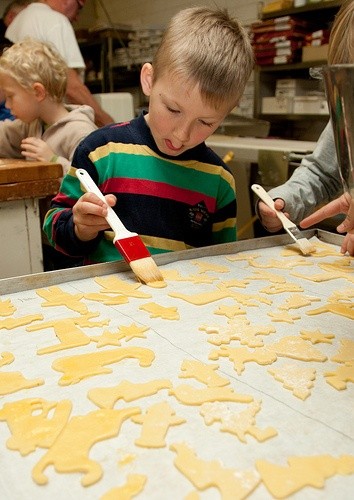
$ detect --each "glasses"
[305,63,354,79]
[76,0,84,13]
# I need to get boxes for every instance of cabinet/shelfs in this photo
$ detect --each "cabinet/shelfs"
[73,23,150,118]
[252,0,346,119]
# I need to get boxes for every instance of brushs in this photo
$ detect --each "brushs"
[76,168,163,284]
[252,184,317,255]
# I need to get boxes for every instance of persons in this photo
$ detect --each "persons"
[300,193,354,233]
[0,42,98,175]
[256,0,354,258]
[0,0,116,127]
[44,8,254,266]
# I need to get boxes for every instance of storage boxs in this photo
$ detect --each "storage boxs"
[247,0,338,116]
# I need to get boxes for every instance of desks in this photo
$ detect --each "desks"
[205,134,320,161]
